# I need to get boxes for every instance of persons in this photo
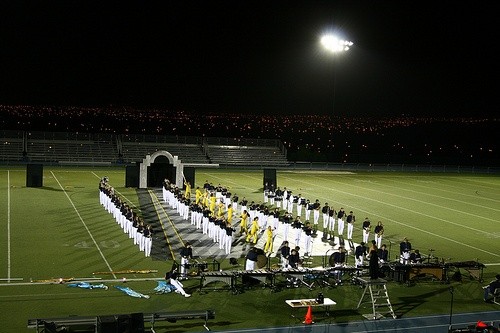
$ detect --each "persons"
[0,104,499,166]
[482,274,500,301]
[99,175,153,257]
[162,179,421,297]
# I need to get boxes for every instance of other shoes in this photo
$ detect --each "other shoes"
[186,278,188,280]
[185,294,191,297]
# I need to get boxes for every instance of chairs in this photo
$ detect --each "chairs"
[488,288,500,305]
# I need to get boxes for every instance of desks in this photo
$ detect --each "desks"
[27,310,215,333]
[173,257,487,295]
[284,298,337,324]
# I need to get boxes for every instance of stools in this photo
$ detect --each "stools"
[0,130,291,168]
